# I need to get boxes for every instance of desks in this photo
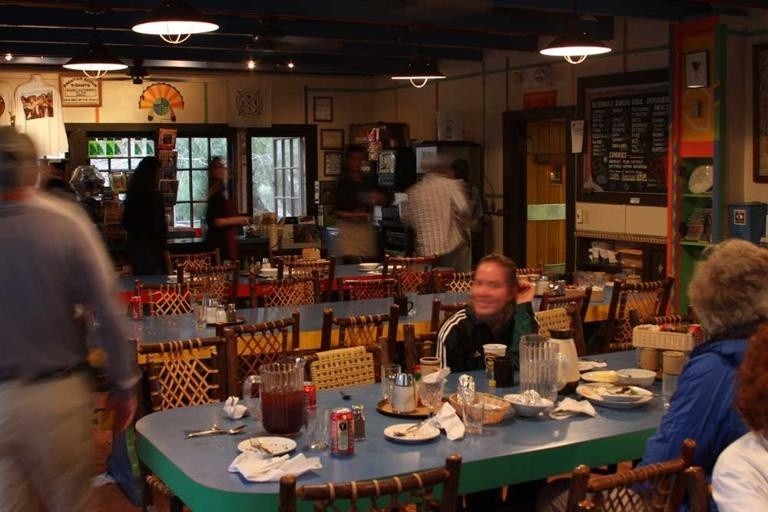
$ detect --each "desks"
[133,350,719,509]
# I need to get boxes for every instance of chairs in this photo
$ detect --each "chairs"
[318,302,400,363]
[402,322,438,372]
[127,326,239,511]
[111,247,546,318]
[628,307,699,334]
[279,452,463,511]
[530,302,587,359]
[539,285,594,328]
[607,274,675,352]
[540,438,698,512]
[216,311,303,400]
[685,463,719,511]
[292,335,390,389]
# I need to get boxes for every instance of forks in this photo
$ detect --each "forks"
[249,437,272,455]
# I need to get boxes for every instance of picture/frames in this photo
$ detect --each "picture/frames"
[680,48,709,90]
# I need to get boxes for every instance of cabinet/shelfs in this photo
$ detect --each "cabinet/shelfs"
[679,191,712,249]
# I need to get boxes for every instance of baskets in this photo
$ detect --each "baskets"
[633,324,707,352]
[303,248,320,261]
[449,392,510,424]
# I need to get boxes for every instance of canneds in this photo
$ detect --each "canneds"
[687,324,704,345]
[130,295,143,320]
[330,408,355,454]
[304,381,317,416]
[132,279,142,296]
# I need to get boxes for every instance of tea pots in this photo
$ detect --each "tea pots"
[391,372,416,415]
[516,338,559,402]
[243,355,306,438]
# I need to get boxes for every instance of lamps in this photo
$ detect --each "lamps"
[390,52,446,89]
[62,23,129,80]
[129,4,219,45]
[539,27,613,67]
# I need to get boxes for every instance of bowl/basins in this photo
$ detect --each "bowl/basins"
[616,367,658,387]
[168,275,186,280]
[260,268,278,274]
[504,393,553,418]
[359,262,380,268]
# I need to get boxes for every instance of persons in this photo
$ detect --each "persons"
[535,238,768,512]
[710,322,768,512]
[324,144,483,273]
[120,158,169,275]
[0,126,143,512]
[198,155,253,266]
[433,253,547,512]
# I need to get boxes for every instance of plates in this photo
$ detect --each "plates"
[576,382,655,410]
[236,436,297,455]
[580,362,597,375]
[165,277,217,284]
[581,370,617,383]
[688,164,713,195]
[384,422,441,442]
[257,274,276,277]
[358,269,381,271]
[376,265,406,270]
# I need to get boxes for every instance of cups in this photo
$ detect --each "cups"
[493,355,514,387]
[304,404,329,450]
[519,271,606,295]
[462,395,485,436]
[483,343,506,387]
[418,377,447,409]
[435,110,454,142]
[419,356,441,377]
[380,364,402,400]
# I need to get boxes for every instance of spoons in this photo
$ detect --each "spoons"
[183,424,247,439]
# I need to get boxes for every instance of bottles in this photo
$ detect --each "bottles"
[351,405,367,441]
[216,304,228,323]
[227,303,237,322]
[205,301,216,324]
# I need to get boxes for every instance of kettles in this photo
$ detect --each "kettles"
[547,326,580,393]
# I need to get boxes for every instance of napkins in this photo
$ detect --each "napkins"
[426,402,466,441]
[223,394,247,421]
[230,451,325,482]
[549,396,596,421]
